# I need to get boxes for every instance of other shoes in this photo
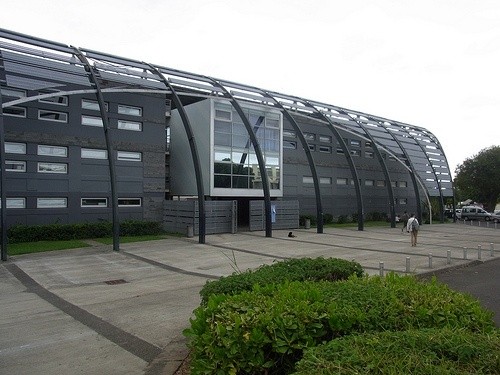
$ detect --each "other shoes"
[410,245,413,247]
[414,244,417,247]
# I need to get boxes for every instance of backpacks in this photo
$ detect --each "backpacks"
[409,217,419,231]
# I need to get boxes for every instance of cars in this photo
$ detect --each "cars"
[444,208,454,218]
[490,210,500,223]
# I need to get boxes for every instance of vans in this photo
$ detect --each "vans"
[461,206,492,221]
[451,209,462,221]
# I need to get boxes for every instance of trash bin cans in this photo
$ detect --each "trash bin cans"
[304,218,310,229]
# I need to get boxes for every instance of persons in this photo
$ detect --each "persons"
[407,214,419,247]
[395,214,402,223]
[401,212,410,233]
